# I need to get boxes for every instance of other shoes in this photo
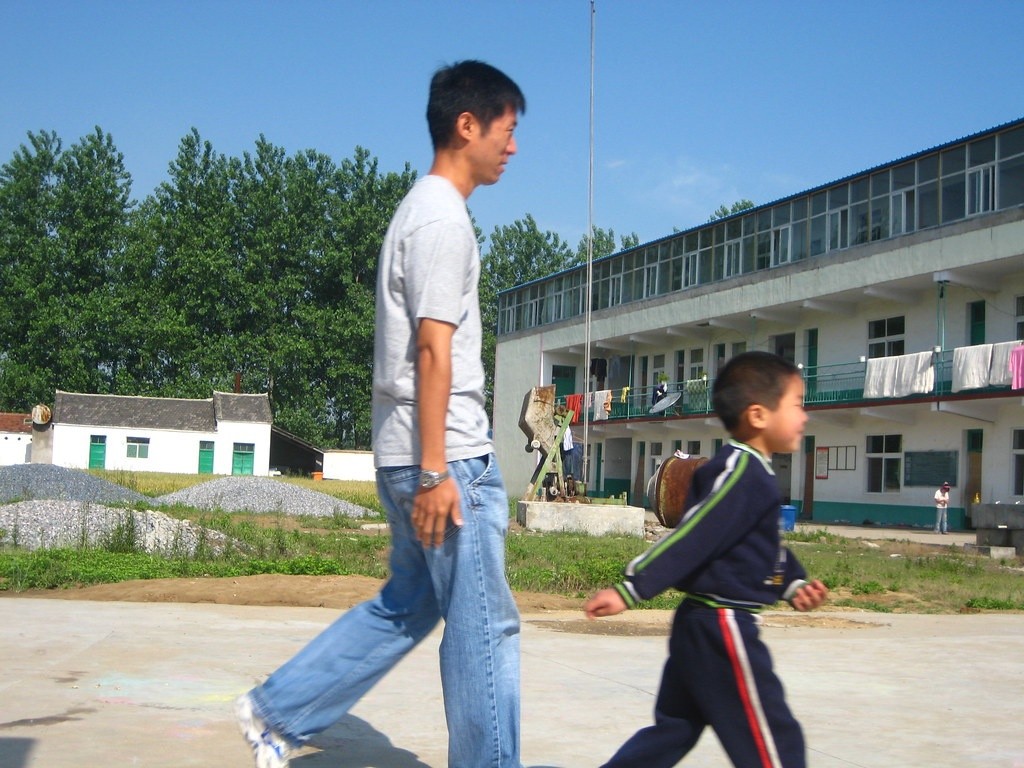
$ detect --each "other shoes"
[234,693,297,768]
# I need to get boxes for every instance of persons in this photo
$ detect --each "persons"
[584,351,829,768]
[235,60,562,768]
[934,482,950,535]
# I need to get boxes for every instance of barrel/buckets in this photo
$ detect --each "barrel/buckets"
[780,506,797,531]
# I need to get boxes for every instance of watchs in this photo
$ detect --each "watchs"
[417,469,450,488]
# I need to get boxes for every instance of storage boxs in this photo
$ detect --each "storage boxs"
[311,472,323,480]
